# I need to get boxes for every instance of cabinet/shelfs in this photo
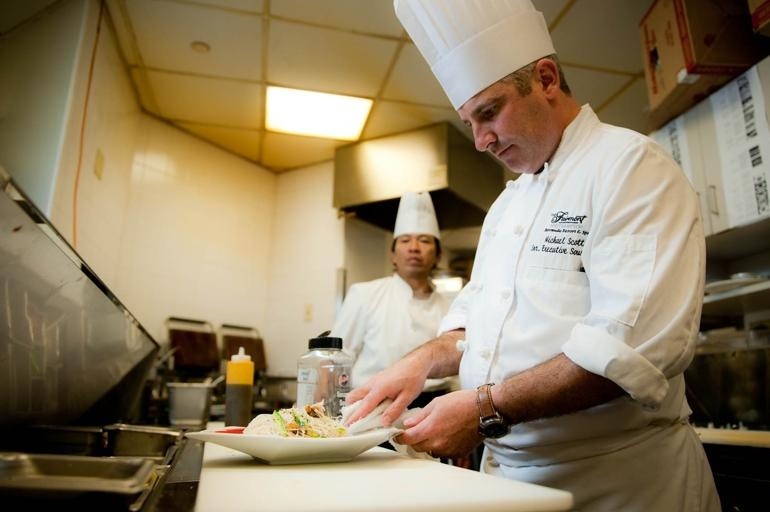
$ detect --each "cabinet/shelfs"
[685,280,770,448]
[646,57,770,259]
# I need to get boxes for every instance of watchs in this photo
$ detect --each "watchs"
[476,383,509,439]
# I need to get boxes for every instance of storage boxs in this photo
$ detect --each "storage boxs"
[637,0,770,125]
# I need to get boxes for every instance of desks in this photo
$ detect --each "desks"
[193,421,575,512]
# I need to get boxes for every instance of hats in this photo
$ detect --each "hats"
[392,190,440,240]
[393,0,556,109]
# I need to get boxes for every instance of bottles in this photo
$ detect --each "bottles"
[296,331,352,421]
[224,346,255,425]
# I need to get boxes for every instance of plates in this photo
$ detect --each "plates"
[184,425,403,466]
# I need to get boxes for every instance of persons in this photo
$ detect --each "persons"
[315,191,472,471]
[343,0,724,512]
